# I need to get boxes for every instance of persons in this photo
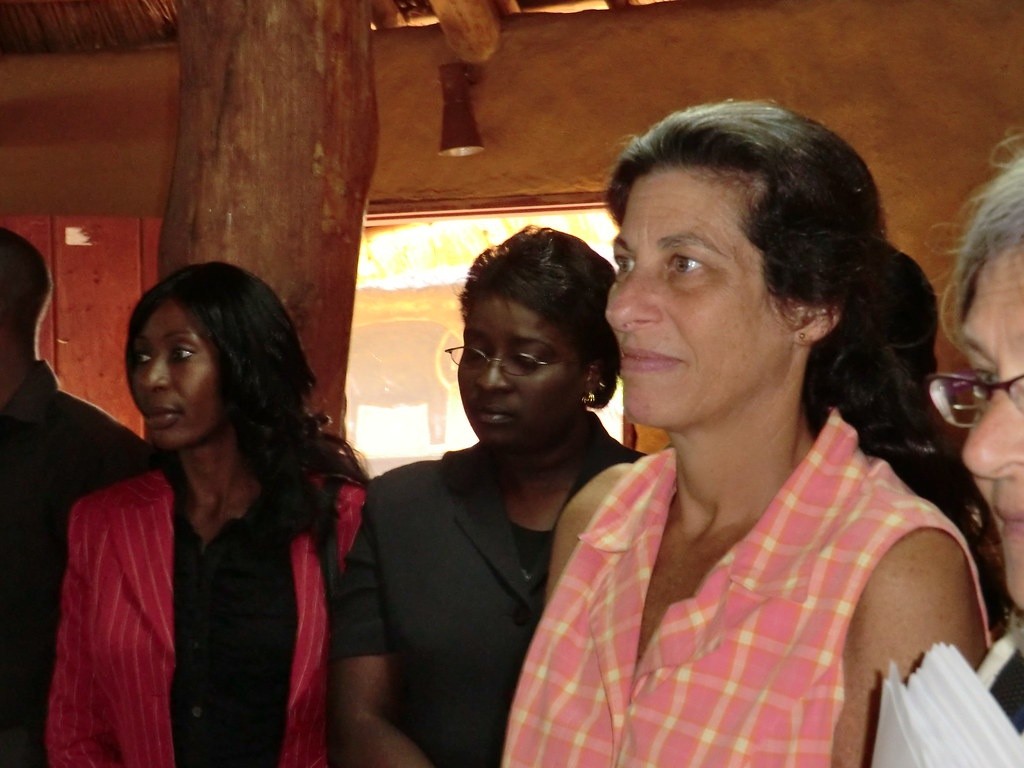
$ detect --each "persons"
[44,262,368,768]
[956,159,1024,744]
[333,226,645,768]
[1,225,149,768]
[501,104,1014,768]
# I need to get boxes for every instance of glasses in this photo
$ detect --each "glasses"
[445,347,562,376]
[928,373,1024,428]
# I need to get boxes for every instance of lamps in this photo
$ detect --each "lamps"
[439,62,485,158]
